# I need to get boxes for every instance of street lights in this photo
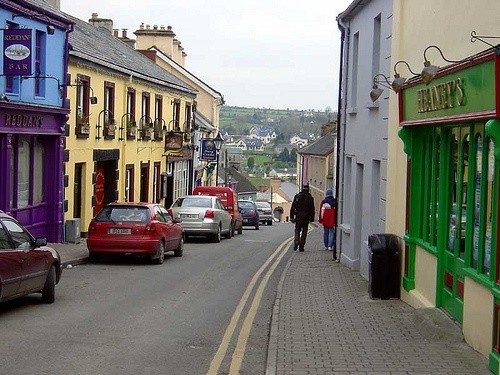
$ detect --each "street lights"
[213,131,225,187]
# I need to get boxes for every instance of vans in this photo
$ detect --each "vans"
[192,186,243,236]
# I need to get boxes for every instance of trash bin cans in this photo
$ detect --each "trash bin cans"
[366,232,402,301]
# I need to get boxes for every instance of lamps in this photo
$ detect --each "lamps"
[13,14,55,35]
[60,84,97,105]
[212,132,224,150]
[96,110,197,142]
[370,46,473,102]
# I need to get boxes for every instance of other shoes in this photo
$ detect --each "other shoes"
[294,244,298,251]
[328,246,332,251]
[299,249,304,251]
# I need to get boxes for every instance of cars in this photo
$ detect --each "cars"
[255,202,273,226]
[0,210,63,303]
[87,202,185,265]
[166,195,233,242]
[237,199,260,230]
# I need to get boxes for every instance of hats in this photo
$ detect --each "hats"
[326,188,332,196]
[303,184,309,190]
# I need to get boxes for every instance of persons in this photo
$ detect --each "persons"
[289,185,315,251]
[318,186,336,251]
